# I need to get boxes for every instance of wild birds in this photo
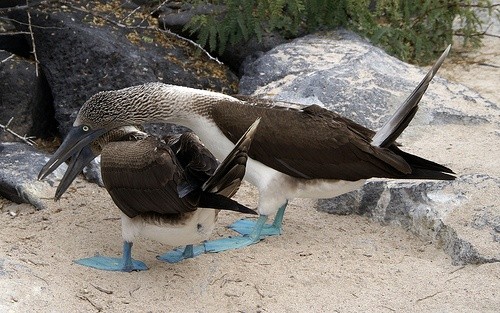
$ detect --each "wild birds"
[34,43,457,266]
[52,116,262,272]
